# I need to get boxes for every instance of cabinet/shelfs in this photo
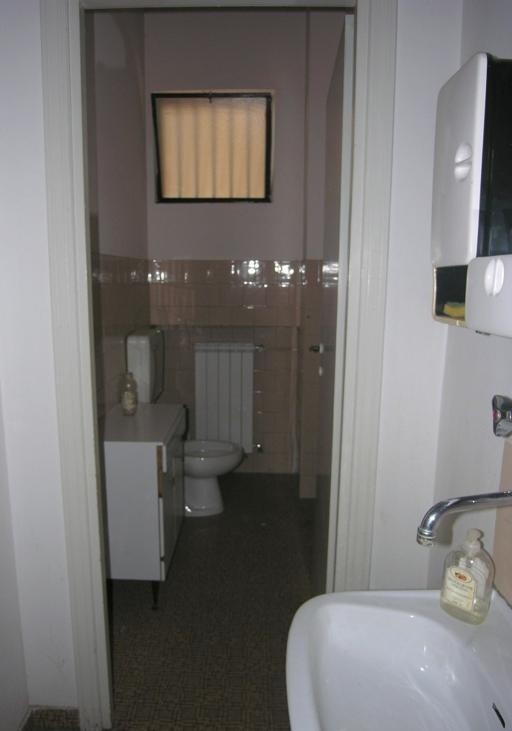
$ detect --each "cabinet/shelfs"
[104,402,186,582]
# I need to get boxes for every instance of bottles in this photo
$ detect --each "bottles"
[119,371,139,415]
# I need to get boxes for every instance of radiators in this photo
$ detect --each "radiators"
[195,342,265,454]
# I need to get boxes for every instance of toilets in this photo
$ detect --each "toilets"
[126,323,244,518]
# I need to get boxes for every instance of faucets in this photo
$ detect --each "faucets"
[415,490,512,548]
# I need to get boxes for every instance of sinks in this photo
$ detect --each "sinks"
[284,589,512,731]
